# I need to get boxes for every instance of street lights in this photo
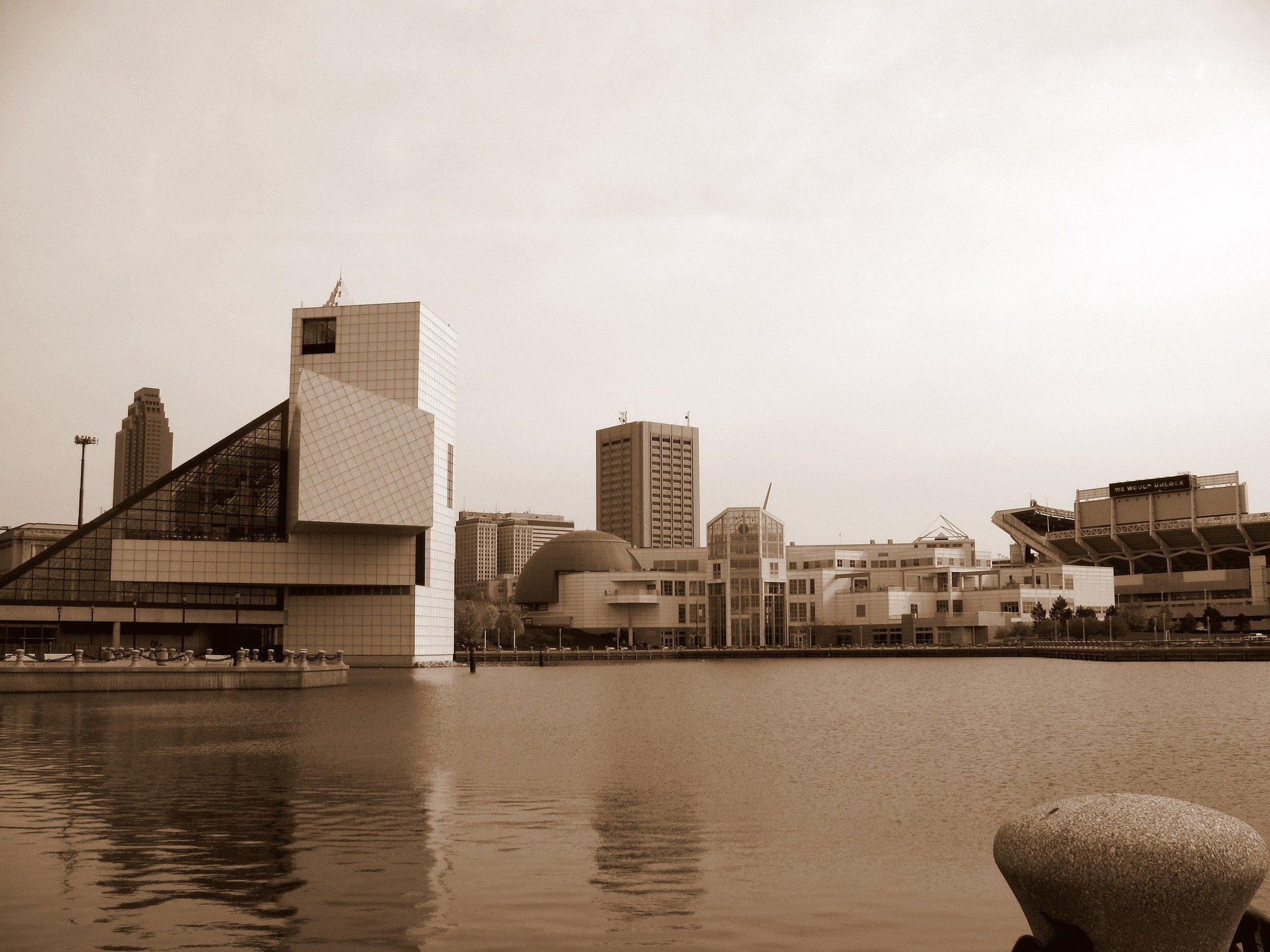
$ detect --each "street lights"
[466,600,474,609]
[1154,618,1157,641]
[73,435,101,530]
[1108,616,1112,641]
[497,628,500,650]
[740,628,743,649]
[859,626,863,648]
[672,629,675,650]
[1162,613,1166,640]
[913,625,916,648]
[559,628,562,652]
[1081,618,1086,642]
[1054,621,1058,642]
[1207,616,1210,640]
[616,628,620,652]
[808,627,812,649]
[1066,620,1070,642]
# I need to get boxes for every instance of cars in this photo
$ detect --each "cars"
[1245,633,1268,640]
